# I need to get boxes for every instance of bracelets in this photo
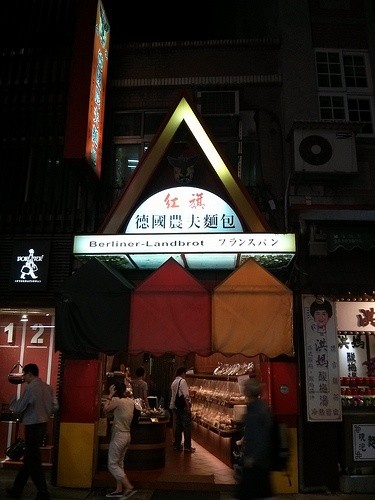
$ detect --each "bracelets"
[107,397,111,401]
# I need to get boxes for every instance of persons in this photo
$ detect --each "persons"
[7,362,59,500]
[239,379,280,500]
[169,366,196,452]
[103,380,136,500]
[130,365,150,408]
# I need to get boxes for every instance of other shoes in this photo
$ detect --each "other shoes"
[174,446,183,449]
[6,488,20,498]
[185,448,195,451]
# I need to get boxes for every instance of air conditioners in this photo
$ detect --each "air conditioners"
[197,90,240,117]
[290,129,360,180]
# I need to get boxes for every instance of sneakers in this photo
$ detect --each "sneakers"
[119,488,137,500]
[105,490,123,498]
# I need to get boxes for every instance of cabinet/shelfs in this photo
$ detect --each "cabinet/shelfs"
[185,371,257,469]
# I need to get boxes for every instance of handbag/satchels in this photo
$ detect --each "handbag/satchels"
[175,394,186,408]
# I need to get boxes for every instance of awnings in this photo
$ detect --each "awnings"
[325,225,375,253]
[53,257,296,358]
